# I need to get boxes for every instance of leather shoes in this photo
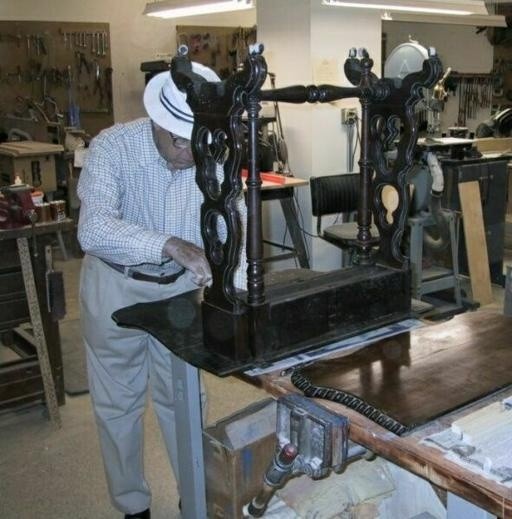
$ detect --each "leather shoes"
[124,509,150,519]
[178,498,182,512]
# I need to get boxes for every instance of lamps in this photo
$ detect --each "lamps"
[321,1,488,17]
[141,2,255,22]
[378,9,508,29]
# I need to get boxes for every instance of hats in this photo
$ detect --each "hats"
[143,61,227,144]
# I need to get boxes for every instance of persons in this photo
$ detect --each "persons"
[76,61,248,518]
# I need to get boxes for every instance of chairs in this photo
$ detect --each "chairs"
[308,172,382,267]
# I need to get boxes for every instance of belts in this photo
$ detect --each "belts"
[101,257,186,285]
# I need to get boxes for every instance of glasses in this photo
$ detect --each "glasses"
[167,132,191,151]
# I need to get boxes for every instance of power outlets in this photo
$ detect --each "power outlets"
[341,105,360,125]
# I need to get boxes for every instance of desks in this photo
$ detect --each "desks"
[1,216,74,430]
[240,167,311,270]
[109,268,511,519]
[1,138,65,195]
